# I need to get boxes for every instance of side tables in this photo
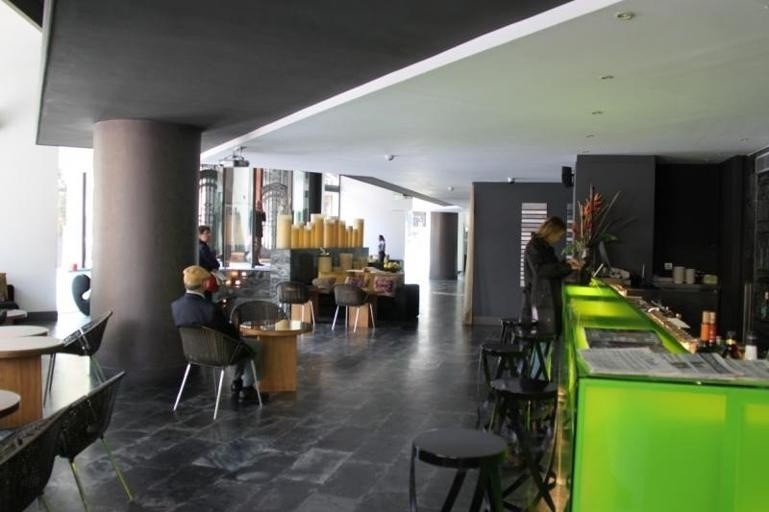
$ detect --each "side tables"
[1,322,65,430]
[238,319,303,393]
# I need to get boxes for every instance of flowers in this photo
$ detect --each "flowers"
[562,182,641,255]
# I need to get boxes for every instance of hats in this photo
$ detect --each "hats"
[183,265,212,286]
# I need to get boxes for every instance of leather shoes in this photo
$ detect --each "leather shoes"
[231,379,270,404]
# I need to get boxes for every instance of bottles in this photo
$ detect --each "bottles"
[721,332,742,358]
[743,334,759,361]
[700,311,717,345]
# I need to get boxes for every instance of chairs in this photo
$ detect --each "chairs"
[1,403,69,512]
[209,268,289,305]
[171,325,263,420]
[54,368,135,511]
[40,309,114,407]
[226,299,288,326]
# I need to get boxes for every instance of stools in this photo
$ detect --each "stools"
[405,316,566,510]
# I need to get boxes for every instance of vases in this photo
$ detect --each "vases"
[578,245,595,269]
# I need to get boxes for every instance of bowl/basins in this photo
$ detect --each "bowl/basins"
[672,266,696,285]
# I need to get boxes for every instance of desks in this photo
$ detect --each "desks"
[305,280,420,331]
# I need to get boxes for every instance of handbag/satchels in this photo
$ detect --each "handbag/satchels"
[205,273,219,293]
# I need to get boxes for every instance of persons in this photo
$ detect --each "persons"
[170,266,271,401]
[521,215,579,377]
[249,200,267,265]
[377,234,386,263]
[198,225,220,272]
